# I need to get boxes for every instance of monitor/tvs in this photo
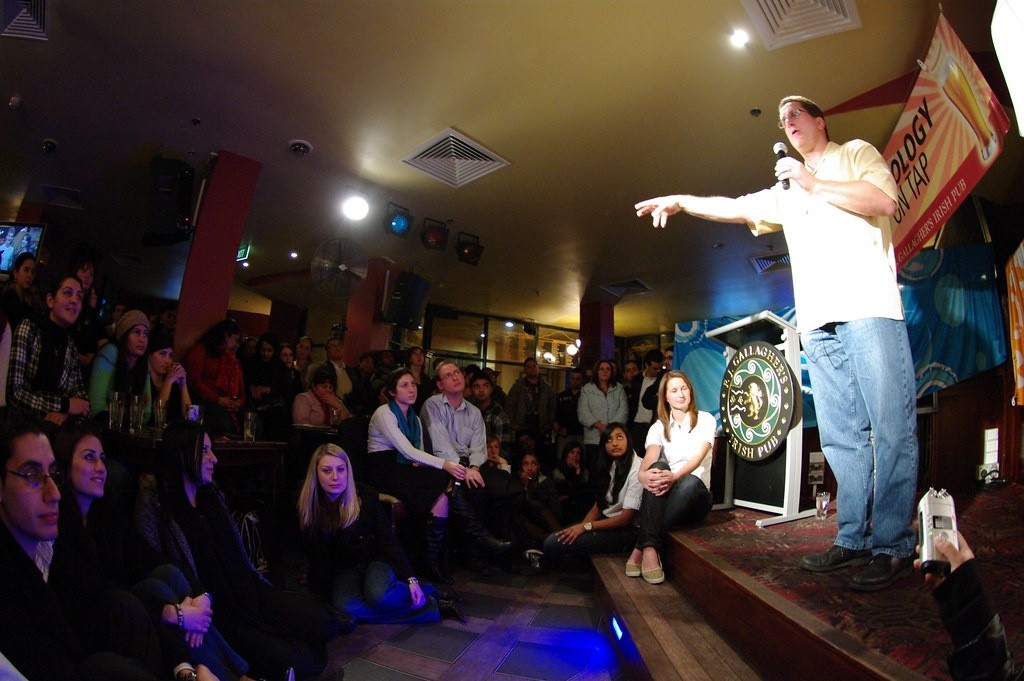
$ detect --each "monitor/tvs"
[0,222,47,282]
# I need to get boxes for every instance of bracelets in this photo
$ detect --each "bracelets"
[175,604,183,626]
[181,671,196,681]
[408,580,418,584]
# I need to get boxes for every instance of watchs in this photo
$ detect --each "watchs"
[584,522,592,531]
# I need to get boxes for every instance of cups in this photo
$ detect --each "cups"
[244,412,255,441]
[185,404,203,424]
[815,492,831,520]
[330,408,341,432]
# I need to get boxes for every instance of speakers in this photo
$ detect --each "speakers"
[141,158,196,247]
[384,270,433,331]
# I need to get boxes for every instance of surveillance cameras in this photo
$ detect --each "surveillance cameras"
[288,139,313,156]
[42,138,59,152]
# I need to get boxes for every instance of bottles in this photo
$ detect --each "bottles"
[152,399,165,439]
[110,391,123,429]
[130,395,144,435]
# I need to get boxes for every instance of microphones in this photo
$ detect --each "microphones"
[773,142,790,190]
[452,456,470,499]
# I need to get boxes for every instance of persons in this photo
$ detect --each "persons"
[0,406,218,681]
[368,346,717,584]
[297,443,467,625]
[135,420,355,681]
[913,518,1024,681]
[635,96,918,592]
[53,425,297,681]
[0,252,396,439]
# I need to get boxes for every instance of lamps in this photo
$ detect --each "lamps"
[420,216,448,252]
[383,201,412,239]
[455,230,483,268]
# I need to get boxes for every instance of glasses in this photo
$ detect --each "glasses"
[1,467,64,488]
[777,108,813,128]
[439,371,461,381]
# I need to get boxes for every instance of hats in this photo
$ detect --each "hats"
[115,309,151,342]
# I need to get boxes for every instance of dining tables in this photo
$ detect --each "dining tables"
[105,427,289,495]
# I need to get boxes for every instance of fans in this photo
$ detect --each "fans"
[309,236,370,299]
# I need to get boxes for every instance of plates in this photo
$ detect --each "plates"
[292,423,330,430]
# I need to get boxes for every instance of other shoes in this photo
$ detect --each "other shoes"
[468,558,497,576]
[642,552,664,584]
[435,590,465,621]
[626,562,641,577]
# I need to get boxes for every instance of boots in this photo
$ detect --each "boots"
[422,511,454,584]
[450,500,513,561]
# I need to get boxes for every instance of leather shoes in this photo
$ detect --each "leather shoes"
[801,545,872,571]
[847,553,915,592]
